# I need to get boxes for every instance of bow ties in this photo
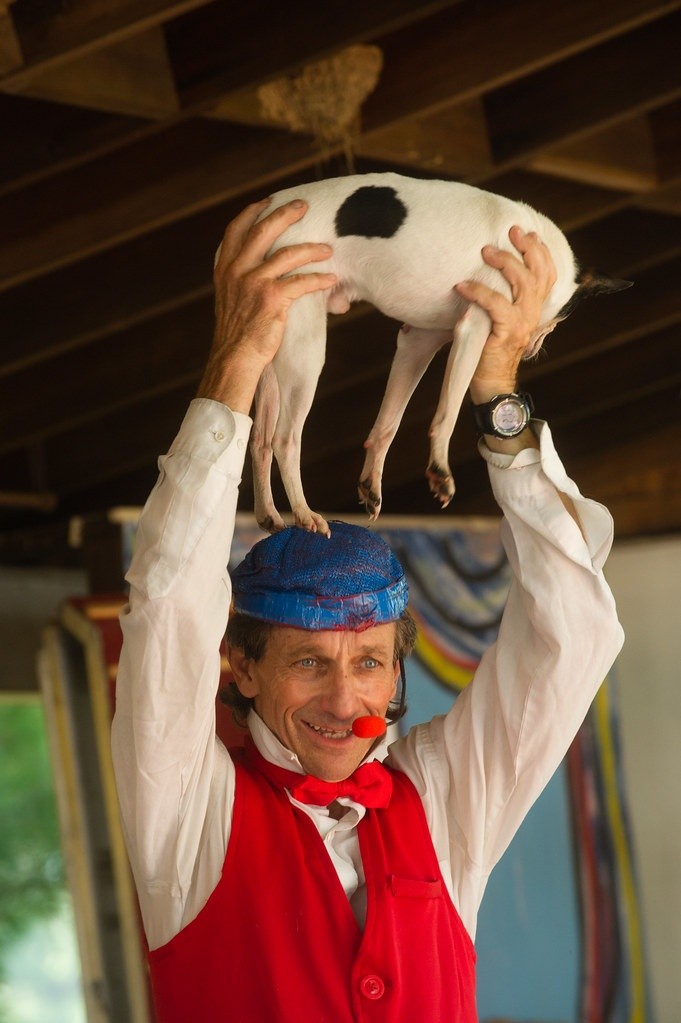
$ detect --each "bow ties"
[291,761,393,809]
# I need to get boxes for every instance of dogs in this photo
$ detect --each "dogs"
[214,170,635,539]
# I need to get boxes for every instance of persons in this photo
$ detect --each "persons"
[110,196,627,1023]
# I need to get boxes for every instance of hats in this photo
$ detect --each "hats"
[231,521,409,631]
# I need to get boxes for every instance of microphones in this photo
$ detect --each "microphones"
[351,657,408,738]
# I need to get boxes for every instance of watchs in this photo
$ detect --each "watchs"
[471,390,535,441]
